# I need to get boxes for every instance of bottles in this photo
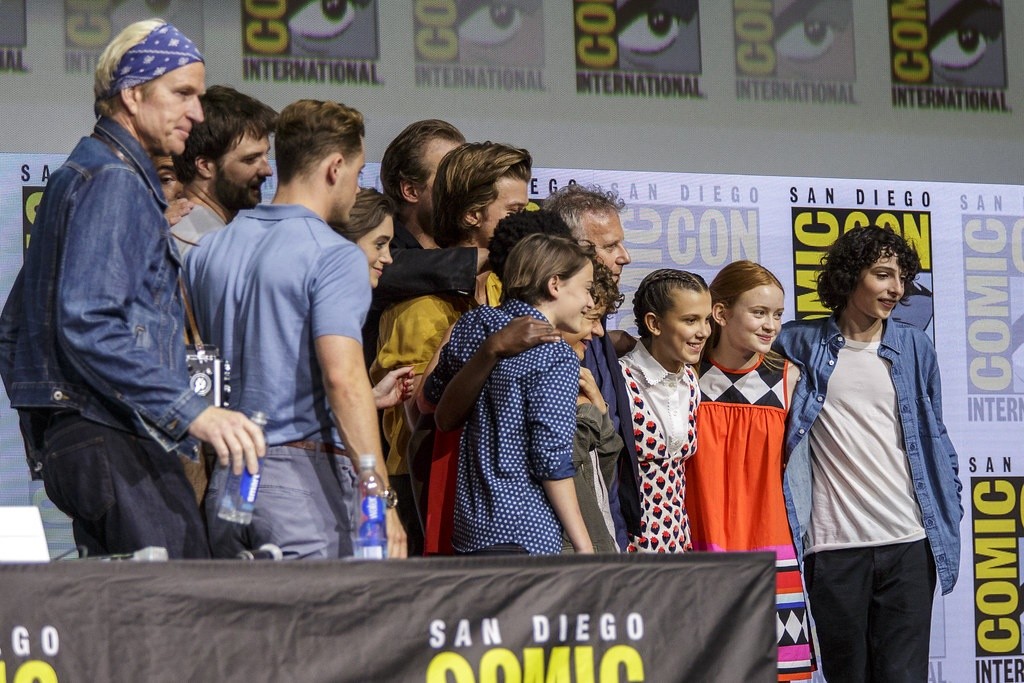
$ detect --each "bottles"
[217,411,268,526]
[349,453,389,560]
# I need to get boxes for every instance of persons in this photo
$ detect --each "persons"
[0,19,265,561]
[685,259,813,683]
[148,84,644,558]
[768,223,965,683]
[179,99,409,558]
[617,268,712,556]
[413,232,596,554]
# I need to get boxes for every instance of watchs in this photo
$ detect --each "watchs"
[385,488,399,508]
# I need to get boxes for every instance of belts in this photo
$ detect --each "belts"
[282,437,348,460]
